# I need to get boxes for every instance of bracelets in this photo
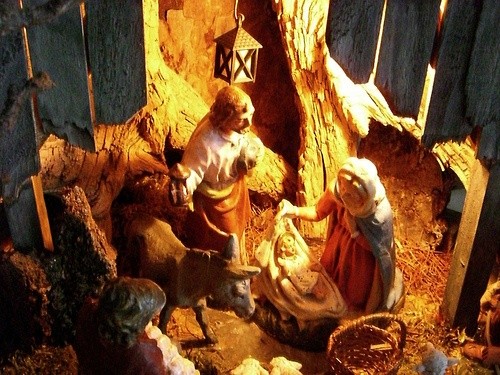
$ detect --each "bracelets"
[293,205,300,220]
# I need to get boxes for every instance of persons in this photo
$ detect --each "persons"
[178,86,259,320]
[274,157,400,320]
[70,276,176,374]
[277,234,326,299]
